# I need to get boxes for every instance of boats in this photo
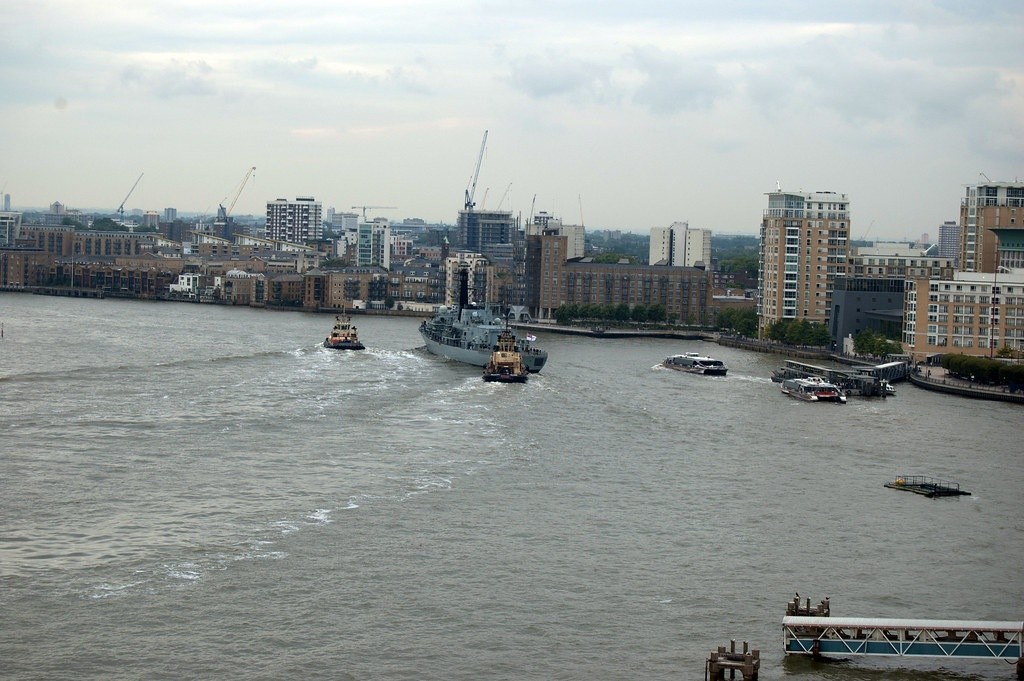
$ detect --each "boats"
[886,473,972,499]
[782,377,847,404]
[879,379,896,396]
[323,306,365,349]
[662,352,727,376]
[484,310,528,383]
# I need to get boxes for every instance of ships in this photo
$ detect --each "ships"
[421,267,547,375]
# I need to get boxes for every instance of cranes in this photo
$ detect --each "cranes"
[351,205,395,221]
[117,171,145,223]
[464,129,540,222]
[224,166,257,220]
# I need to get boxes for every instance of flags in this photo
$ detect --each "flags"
[527,333,537,342]
